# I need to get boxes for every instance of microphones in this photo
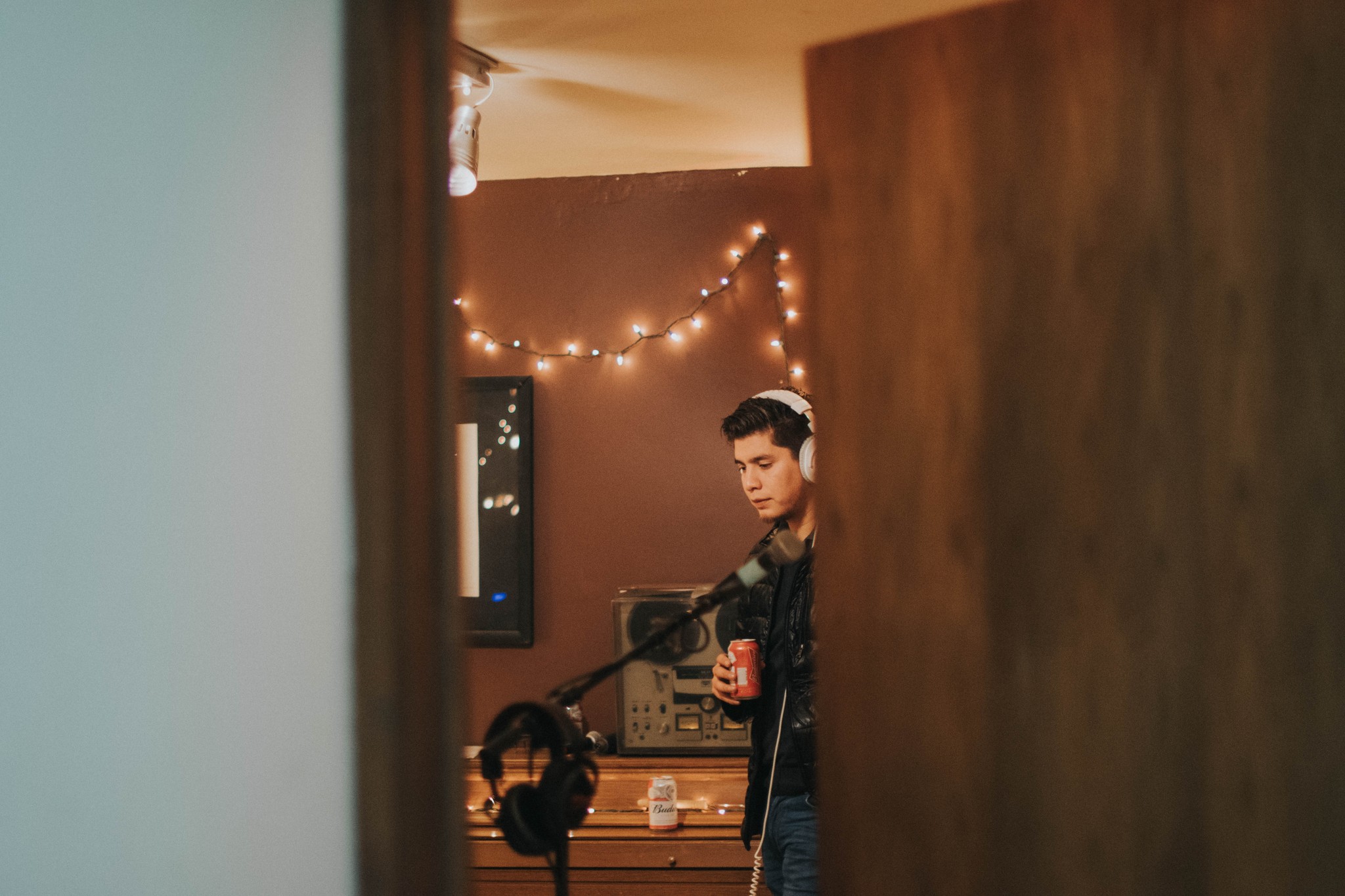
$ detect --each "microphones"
[702,529,807,607]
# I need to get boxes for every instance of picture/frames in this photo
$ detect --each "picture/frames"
[455,376,535,646]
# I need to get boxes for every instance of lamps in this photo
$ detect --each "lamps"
[448,72,494,196]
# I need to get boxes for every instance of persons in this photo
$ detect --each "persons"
[710,388,816,896]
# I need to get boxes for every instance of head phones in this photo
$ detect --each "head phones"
[481,700,599,858]
[749,389,816,483]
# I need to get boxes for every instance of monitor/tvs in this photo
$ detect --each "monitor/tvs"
[450,374,534,647]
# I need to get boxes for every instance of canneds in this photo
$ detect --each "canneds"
[648,776,678,831]
[727,638,762,700]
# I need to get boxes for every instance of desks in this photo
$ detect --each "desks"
[466,747,772,896]
